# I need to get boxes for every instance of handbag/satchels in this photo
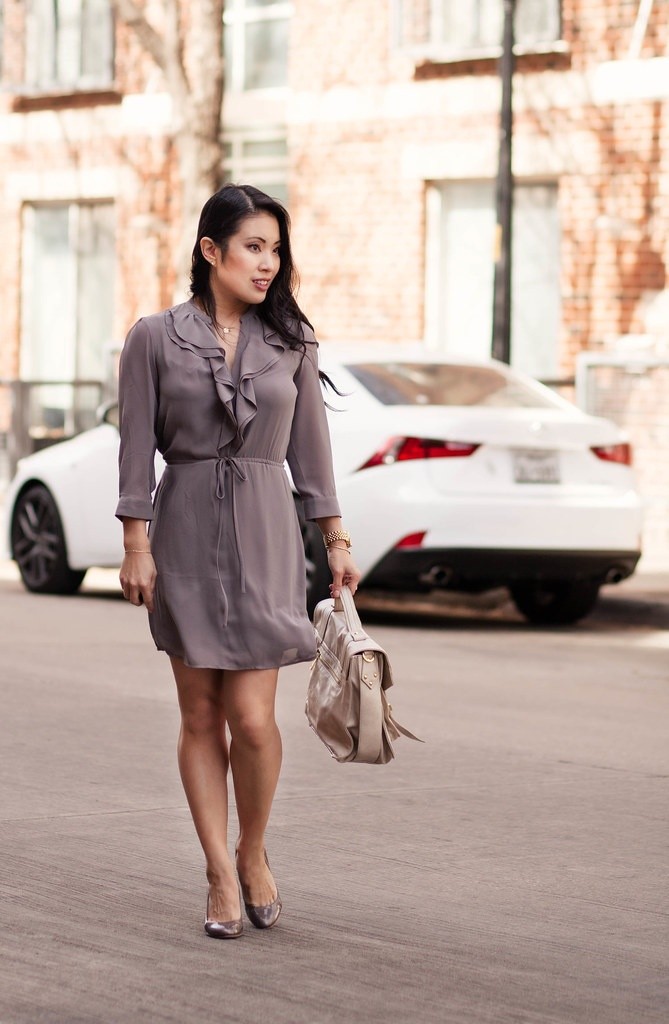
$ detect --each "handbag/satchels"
[305,585,426,763]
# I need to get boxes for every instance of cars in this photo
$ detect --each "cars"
[0,344,643,631]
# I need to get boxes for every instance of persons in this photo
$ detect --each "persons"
[115,183,360,939]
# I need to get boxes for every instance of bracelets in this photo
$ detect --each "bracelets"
[322,531,352,554]
[125,550,152,553]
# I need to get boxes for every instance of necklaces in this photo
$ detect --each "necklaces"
[222,319,236,333]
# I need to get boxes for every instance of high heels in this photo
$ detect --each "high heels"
[234,837,282,928]
[205,888,242,938]
